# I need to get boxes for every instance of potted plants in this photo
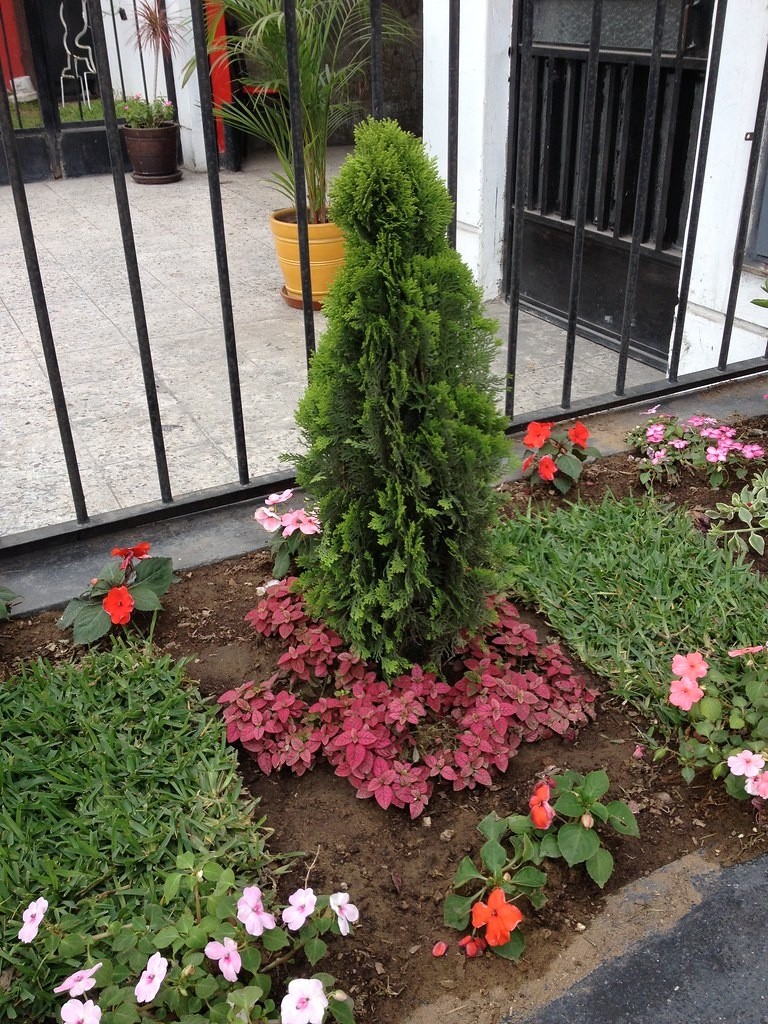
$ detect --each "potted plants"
[180,0,419,312]
[117,92,183,185]
